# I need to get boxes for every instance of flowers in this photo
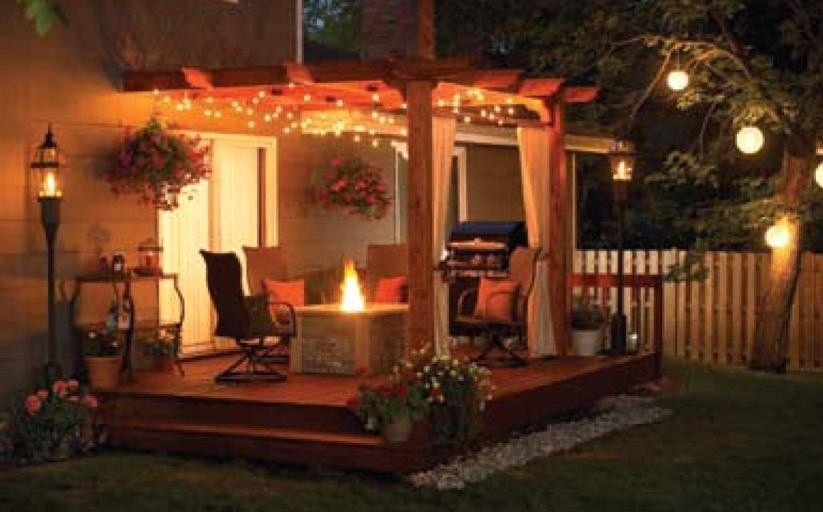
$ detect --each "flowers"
[80,319,120,356]
[346,341,498,420]
[308,156,392,223]
[2,377,100,464]
[105,121,209,209]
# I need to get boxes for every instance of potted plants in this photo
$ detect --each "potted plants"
[569,297,606,358]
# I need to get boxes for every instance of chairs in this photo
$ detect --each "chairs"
[448,242,543,369]
[199,243,409,385]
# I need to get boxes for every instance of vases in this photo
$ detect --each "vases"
[381,412,411,441]
[81,355,122,390]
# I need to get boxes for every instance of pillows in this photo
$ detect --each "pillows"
[470,276,520,324]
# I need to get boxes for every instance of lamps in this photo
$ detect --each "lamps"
[28,121,69,384]
[605,136,642,356]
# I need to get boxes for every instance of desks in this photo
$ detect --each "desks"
[67,270,186,385]
